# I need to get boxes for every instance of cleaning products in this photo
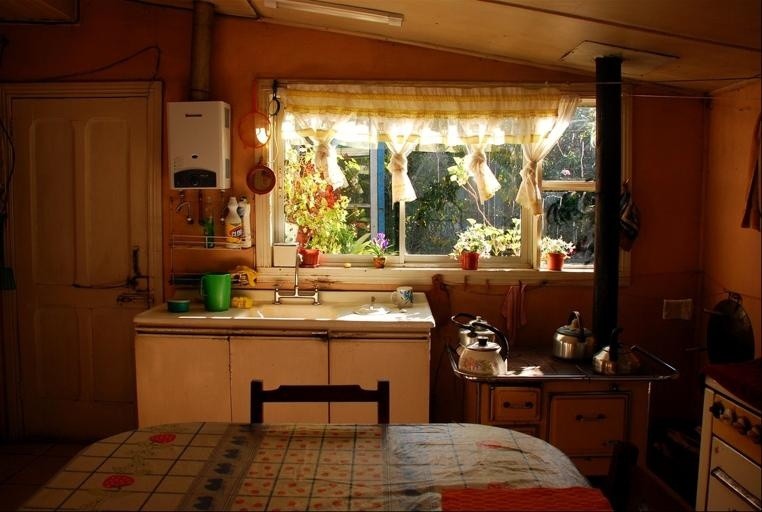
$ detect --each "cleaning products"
[238,196,252,248]
[225,197,242,249]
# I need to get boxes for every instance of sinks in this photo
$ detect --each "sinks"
[240,303,337,320]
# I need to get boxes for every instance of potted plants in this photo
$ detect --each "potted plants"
[539,234,577,270]
[448,233,486,270]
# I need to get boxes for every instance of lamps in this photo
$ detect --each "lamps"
[263,0,403,27]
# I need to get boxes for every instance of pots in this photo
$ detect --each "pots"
[705,292,755,365]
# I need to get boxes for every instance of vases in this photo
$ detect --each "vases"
[303,249,320,267]
[373,258,385,268]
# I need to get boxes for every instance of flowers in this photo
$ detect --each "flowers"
[365,232,394,257]
[278,151,352,254]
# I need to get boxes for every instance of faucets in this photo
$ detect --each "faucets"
[293,254,303,286]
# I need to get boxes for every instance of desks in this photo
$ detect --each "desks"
[16,423,594,511]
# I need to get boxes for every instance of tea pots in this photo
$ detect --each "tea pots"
[552,310,595,360]
[592,327,641,376]
[450,312,511,376]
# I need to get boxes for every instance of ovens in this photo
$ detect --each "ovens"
[695,388,762,511]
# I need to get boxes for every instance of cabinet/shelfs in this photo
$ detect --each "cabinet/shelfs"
[133,327,432,427]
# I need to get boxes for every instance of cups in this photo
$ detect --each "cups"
[390,286,413,308]
